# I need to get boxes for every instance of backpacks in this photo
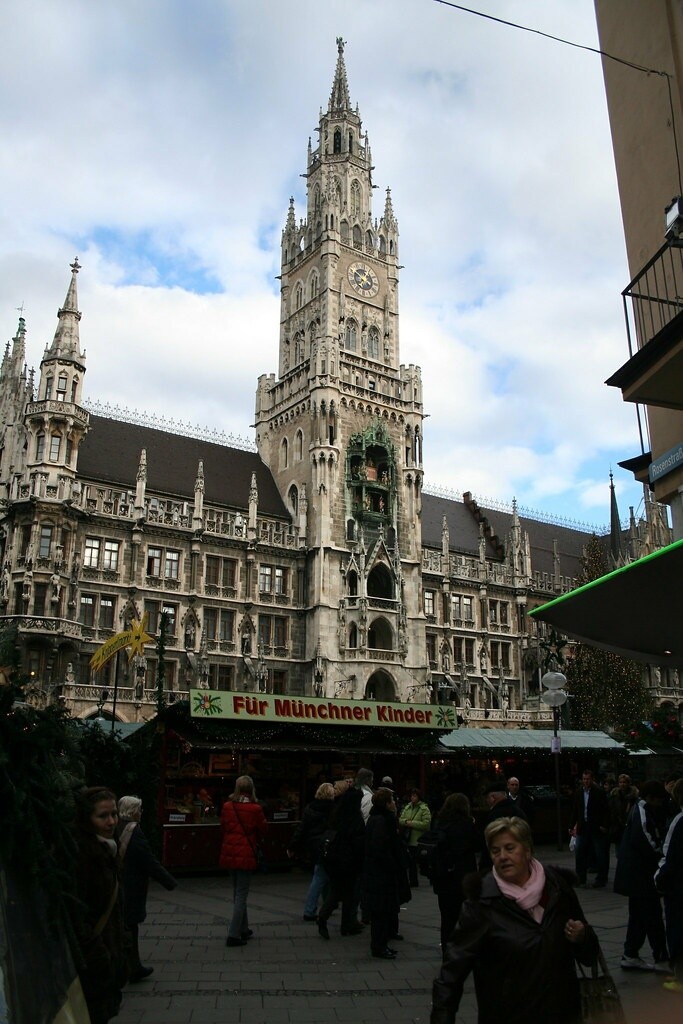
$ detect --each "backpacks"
[415,829,459,876]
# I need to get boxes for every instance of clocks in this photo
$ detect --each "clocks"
[347,260,380,298]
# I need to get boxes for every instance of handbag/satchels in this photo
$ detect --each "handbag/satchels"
[569,830,577,852]
[255,847,265,871]
[400,826,412,852]
[576,950,626,1024]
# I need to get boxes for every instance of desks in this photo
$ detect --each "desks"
[163,821,301,877]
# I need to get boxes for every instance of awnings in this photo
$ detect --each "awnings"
[527,537,683,669]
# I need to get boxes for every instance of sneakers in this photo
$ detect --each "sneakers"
[655,960,675,972]
[621,954,655,972]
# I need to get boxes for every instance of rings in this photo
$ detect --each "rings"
[569,927,572,931]
[567,932,571,936]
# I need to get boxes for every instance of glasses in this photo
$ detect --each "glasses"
[139,809,143,812]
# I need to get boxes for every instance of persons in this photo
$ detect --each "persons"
[53,767,683,1024]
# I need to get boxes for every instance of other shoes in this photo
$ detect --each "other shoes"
[663,982,683,993]
[575,872,587,887]
[129,964,154,984]
[410,882,419,888]
[374,950,396,959]
[242,929,255,938]
[386,946,398,954]
[355,923,365,929]
[391,934,403,940]
[341,928,362,936]
[317,920,330,939]
[666,975,681,981]
[592,874,608,888]
[303,915,319,921]
[226,936,247,947]
